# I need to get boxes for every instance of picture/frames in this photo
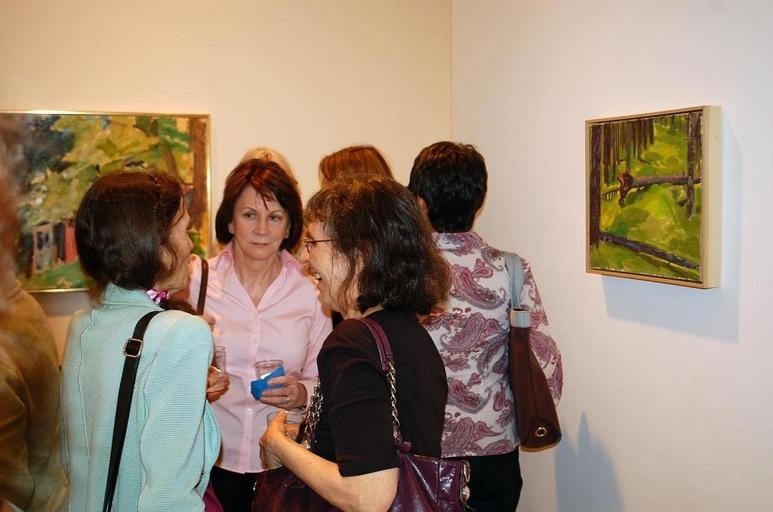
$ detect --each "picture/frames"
[1,110,214,295]
[584,104,722,290]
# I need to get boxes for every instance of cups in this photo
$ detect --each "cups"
[213,345,225,375]
[266,410,303,443]
[253,359,285,379]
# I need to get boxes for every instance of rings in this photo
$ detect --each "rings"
[285,395,291,403]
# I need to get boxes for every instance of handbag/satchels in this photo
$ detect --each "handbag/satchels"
[502,248,563,453]
[245,317,470,511]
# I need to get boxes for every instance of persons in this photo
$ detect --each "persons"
[58,166,229,511]
[260,174,450,512]
[188,158,332,511]
[0,165,72,512]
[409,142,563,511]
[318,145,394,328]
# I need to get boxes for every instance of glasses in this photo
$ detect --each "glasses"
[300,234,339,252]
